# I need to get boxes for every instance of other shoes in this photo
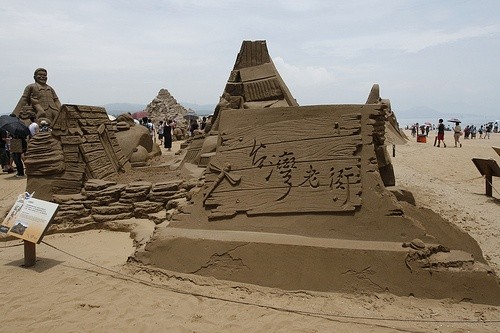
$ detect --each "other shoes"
[8,168,16,174]
[437,145,440,147]
[15,174,24,178]
[444,145,446,147]
[460,144,462,147]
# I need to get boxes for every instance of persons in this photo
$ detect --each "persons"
[464,121,498,139]
[0,130,27,178]
[23,68,61,122]
[202,117,207,129]
[436,119,446,147]
[452,122,462,147]
[407,123,435,137]
[28,116,39,137]
[140,116,176,151]
[185,119,199,137]
[40,121,48,132]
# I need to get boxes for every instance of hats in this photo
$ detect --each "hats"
[41,120,48,124]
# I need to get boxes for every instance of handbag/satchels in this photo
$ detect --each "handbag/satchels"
[460,131,463,135]
[10,136,23,153]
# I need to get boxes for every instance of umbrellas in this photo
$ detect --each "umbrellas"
[183,114,198,119]
[0,115,30,139]
[447,119,461,123]
